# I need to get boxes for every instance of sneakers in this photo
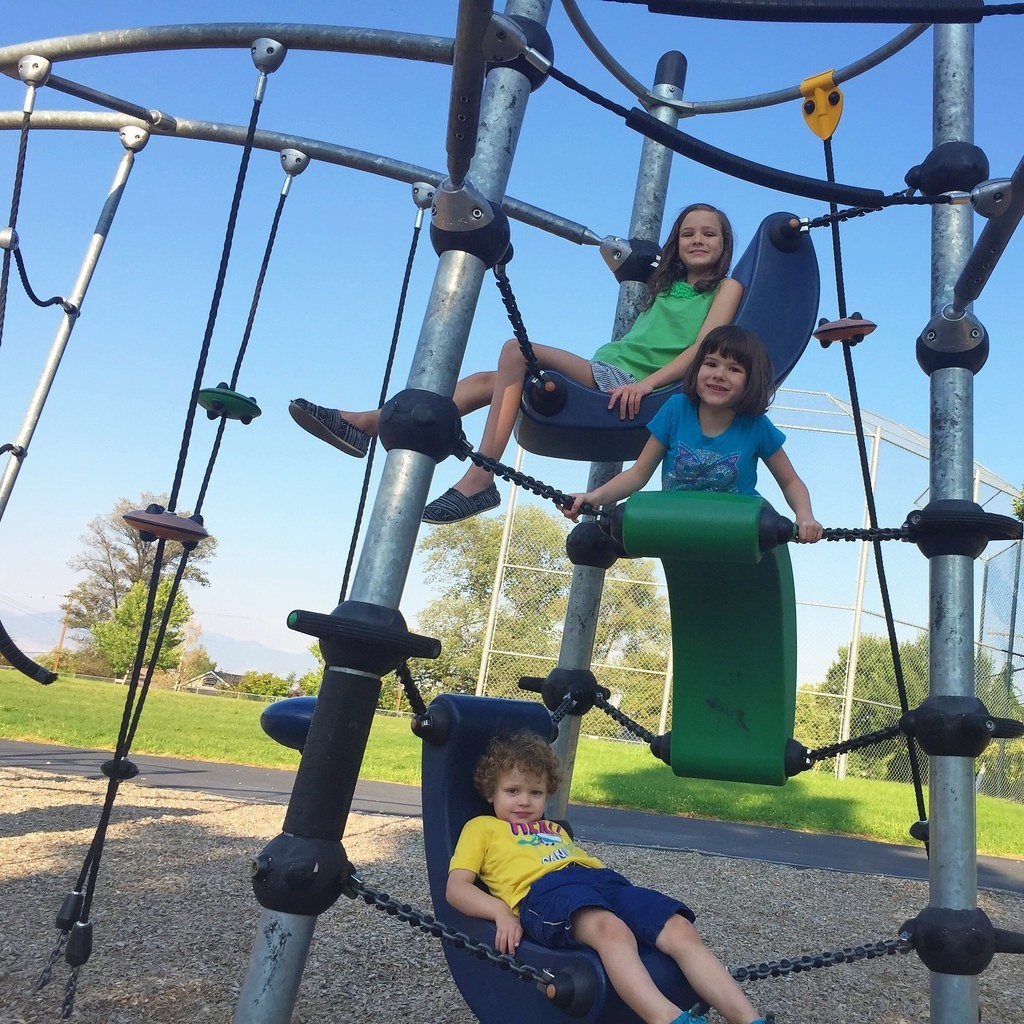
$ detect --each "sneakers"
[751,1019,767,1024]
[670,1011,709,1024]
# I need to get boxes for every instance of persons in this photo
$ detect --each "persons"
[556,325,823,543]
[289,203,744,524]
[446,729,776,1024]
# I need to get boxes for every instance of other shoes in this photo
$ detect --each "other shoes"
[289,398,371,458]
[421,482,502,524]
